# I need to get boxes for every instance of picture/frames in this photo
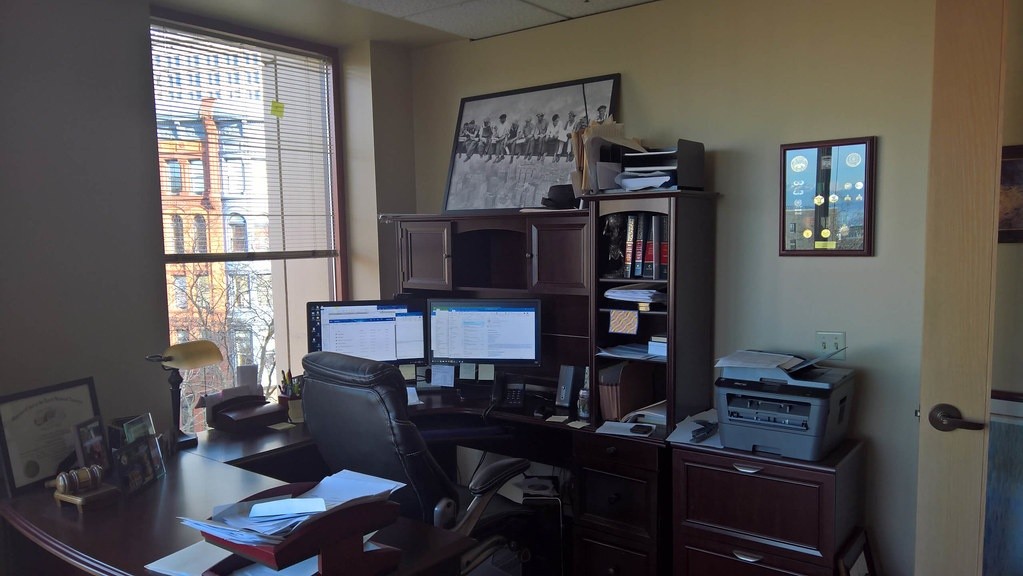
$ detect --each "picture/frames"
[443,72,621,213]
[779,135,877,256]
[0,376,107,499]
[981,389,1023,576]
[998,145,1023,243]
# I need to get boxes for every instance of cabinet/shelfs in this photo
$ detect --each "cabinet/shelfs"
[379,188,714,439]
[671,438,867,576]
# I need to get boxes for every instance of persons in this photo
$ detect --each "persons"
[458,106,607,163]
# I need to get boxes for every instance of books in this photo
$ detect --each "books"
[651,335,667,342]
[622,214,668,278]
[604,284,665,311]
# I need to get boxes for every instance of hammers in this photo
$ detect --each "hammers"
[44,463,104,494]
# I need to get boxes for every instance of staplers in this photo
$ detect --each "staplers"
[689,423,718,443]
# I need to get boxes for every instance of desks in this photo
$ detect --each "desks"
[180,395,670,576]
[0,450,477,576]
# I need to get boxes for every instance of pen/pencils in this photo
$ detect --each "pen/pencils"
[278,368,301,396]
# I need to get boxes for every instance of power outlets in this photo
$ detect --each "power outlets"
[816,331,847,360]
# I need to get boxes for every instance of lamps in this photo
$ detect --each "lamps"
[145,339,224,450]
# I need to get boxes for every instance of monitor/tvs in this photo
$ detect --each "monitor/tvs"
[307,299,428,369]
[427,298,542,390]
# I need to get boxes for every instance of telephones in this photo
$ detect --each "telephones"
[489,370,526,408]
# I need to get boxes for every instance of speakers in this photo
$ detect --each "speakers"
[556,366,575,408]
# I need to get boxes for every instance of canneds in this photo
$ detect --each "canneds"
[578,388,590,420]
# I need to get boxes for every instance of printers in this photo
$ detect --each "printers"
[714,346,857,463]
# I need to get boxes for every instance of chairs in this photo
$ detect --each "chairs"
[302,351,563,576]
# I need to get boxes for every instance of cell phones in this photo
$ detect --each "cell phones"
[630,424,653,434]
